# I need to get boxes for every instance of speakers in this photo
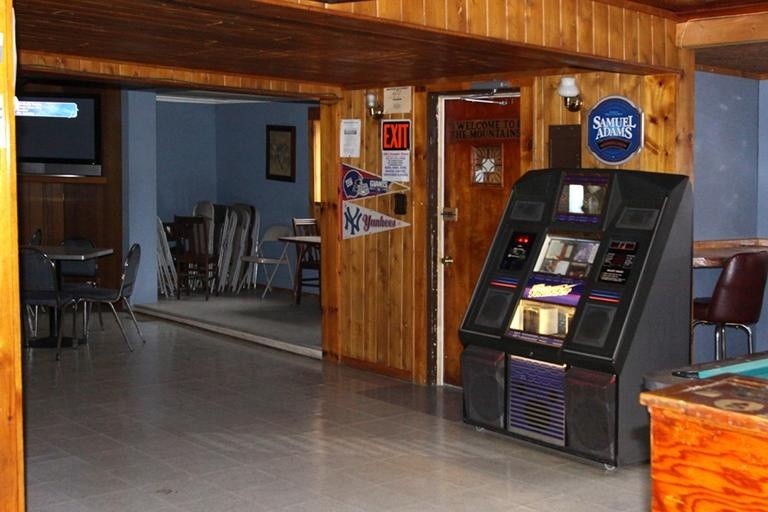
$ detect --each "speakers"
[459,343,507,432]
[562,364,618,468]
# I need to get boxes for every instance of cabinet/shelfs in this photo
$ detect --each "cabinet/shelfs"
[18,171,109,309]
[638,368,767,512]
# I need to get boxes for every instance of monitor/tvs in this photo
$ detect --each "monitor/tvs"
[504,282,585,351]
[533,233,603,282]
[14,95,105,178]
[554,175,612,224]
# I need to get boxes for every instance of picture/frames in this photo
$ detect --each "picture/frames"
[264,123,297,185]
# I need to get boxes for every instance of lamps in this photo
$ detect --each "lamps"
[557,76,585,111]
[366,93,384,118]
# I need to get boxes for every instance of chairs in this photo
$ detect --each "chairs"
[688,249,768,364]
[152,198,322,311]
[18,228,145,364]
